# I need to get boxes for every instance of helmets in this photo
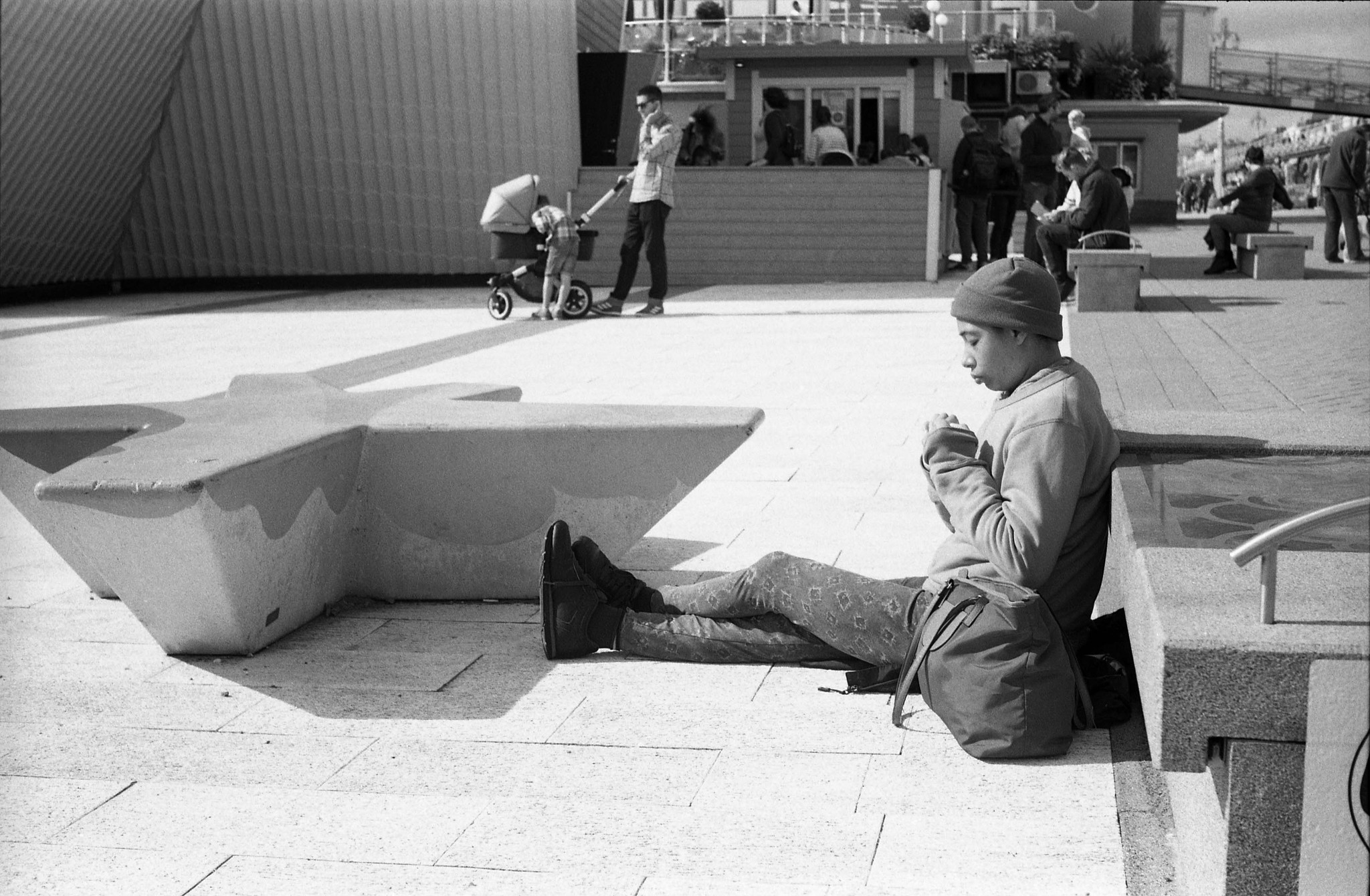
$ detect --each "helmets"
[1111,164,1133,187]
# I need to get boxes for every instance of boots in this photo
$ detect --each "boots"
[1204,251,1237,276]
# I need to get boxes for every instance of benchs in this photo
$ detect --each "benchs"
[1230,232,1313,280]
[1067,249,1150,312]
[0,374,766,657]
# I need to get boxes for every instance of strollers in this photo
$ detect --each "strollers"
[478,174,627,319]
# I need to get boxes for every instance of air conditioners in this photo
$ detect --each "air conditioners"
[1016,71,1052,94]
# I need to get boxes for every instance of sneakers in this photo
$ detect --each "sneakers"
[539,519,612,662]
[574,536,648,610]
[635,298,663,317]
[589,296,624,316]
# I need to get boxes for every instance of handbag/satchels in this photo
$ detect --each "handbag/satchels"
[892,566,1094,759]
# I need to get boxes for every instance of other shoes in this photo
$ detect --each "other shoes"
[1324,255,1344,264]
[1056,276,1076,302]
[950,259,974,273]
[976,260,988,271]
[1351,253,1370,264]
[532,307,563,321]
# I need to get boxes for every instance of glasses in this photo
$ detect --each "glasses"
[635,98,658,109]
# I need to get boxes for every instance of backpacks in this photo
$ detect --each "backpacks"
[963,137,999,183]
[1077,652,1133,727]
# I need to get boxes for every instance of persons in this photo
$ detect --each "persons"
[1191,173,1212,213]
[747,87,804,167]
[676,106,727,167]
[1177,176,1196,213]
[1204,144,1293,275]
[1320,125,1370,265]
[806,104,858,167]
[588,84,684,317]
[532,194,582,321]
[948,93,1134,303]
[885,124,936,168]
[859,141,876,166]
[538,258,1134,671]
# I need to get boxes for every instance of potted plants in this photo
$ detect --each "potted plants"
[969,43,1008,73]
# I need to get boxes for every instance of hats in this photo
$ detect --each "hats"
[689,109,714,126]
[948,255,1068,340]
[1038,92,1063,109]
[1245,145,1264,163]
